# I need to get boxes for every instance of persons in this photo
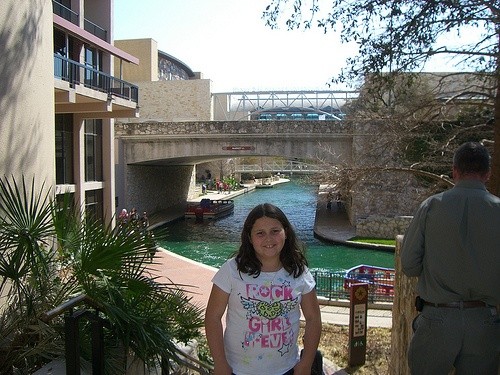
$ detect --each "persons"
[216,179,228,194]
[205,203,322,375]
[400,143,500,375]
[202,185,206,193]
[119,208,149,234]
[145,231,156,262]
[253,175,256,182]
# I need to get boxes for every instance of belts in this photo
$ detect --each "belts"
[427,301,483,308]
